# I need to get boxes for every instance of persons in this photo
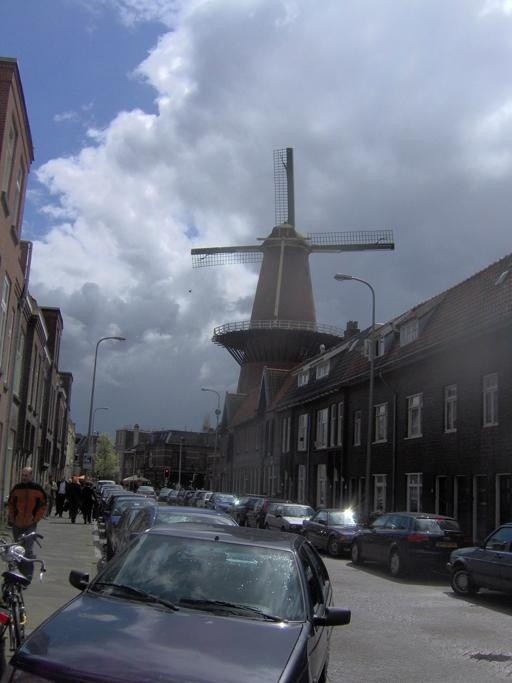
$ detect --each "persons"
[7,465,47,588]
[42,474,98,525]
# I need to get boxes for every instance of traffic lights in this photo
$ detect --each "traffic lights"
[164,468,168,478]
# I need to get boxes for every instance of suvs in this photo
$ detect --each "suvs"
[349,507,467,579]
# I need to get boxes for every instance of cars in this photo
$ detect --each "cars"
[0,522,349,680]
[445,518,512,602]
[93,475,366,573]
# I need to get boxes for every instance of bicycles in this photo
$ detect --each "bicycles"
[0,530,47,675]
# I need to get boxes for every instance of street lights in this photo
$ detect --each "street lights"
[332,272,376,517]
[84,335,128,450]
[177,436,185,488]
[90,402,112,450]
[201,385,221,491]
